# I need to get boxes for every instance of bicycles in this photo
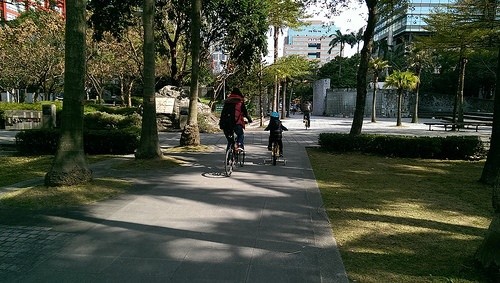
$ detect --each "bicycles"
[301,112,313,130]
[263,129,288,166]
[221,121,253,177]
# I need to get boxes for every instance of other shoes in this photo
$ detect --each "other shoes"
[268,145,272,151]
[238,143,244,150]
[279,151,283,157]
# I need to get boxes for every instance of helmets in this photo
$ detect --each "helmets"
[270,112,279,118]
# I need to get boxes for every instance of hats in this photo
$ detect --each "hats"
[231,87,244,98]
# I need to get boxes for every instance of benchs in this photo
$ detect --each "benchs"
[423,123,487,132]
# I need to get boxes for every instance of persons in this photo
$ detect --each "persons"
[264,112,288,158]
[220,87,253,165]
[300,95,312,128]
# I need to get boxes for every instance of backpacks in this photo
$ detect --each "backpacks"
[218,100,241,130]
